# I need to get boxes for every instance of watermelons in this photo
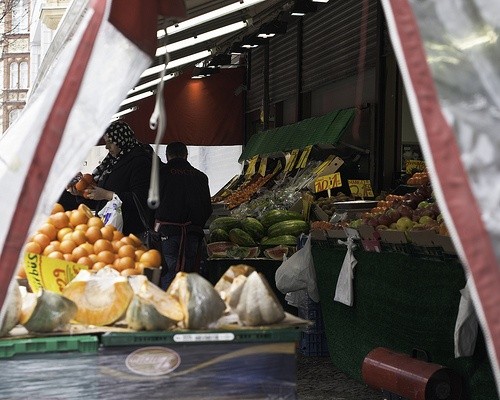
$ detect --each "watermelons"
[206,208,309,260]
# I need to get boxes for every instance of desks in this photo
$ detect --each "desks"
[205,258,285,285]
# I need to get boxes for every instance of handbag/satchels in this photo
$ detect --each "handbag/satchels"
[139,231,169,274]
[98,194,124,233]
[273,234,320,302]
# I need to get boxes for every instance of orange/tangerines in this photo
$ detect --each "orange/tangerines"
[16,202,161,275]
[75,174,96,199]
[370,167,428,214]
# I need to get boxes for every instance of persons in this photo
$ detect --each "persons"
[66,119,162,249]
[155,140,212,292]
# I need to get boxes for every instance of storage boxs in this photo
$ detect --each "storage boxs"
[205,155,345,228]
[298,289,327,360]
[308,186,456,254]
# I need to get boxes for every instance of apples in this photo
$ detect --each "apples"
[375,187,448,235]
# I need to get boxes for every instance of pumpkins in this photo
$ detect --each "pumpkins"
[0,266,286,338]
